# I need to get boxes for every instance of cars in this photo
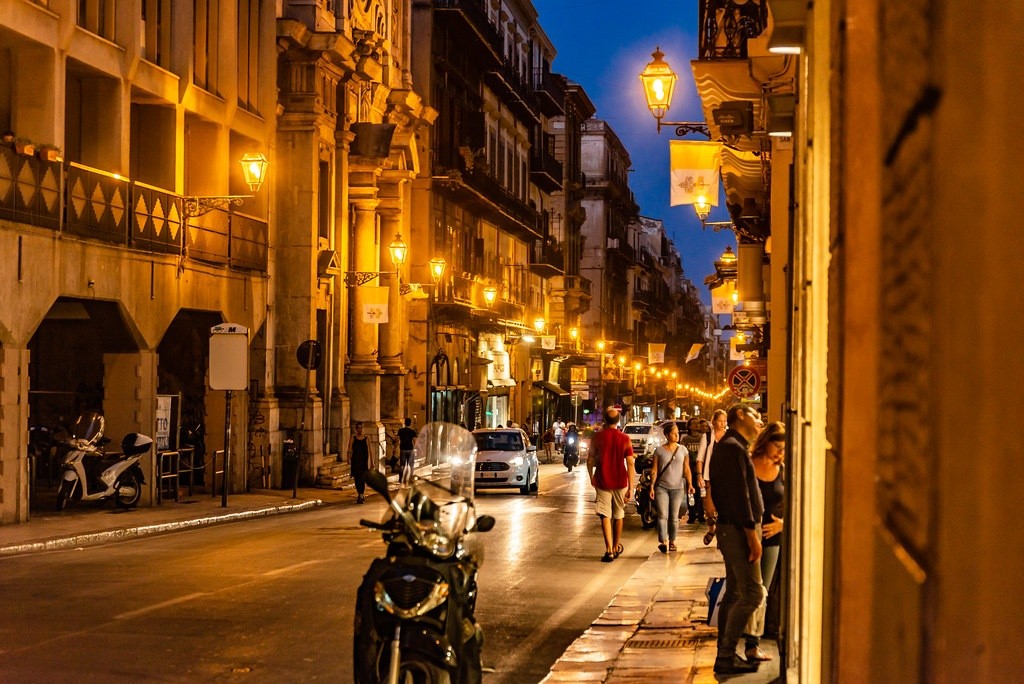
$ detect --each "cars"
[578,426,600,464]
[470,428,540,495]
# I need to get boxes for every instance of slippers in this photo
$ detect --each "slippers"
[703,528,716,545]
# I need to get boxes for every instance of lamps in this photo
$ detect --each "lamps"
[400,249,447,294]
[468,282,497,330]
[765,96,795,137]
[181,146,268,218]
[693,203,732,230]
[341,232,408,288]
[768,27,805,57]
[639,46,708,136]
[509,313,729,399]
[714,244,738,281]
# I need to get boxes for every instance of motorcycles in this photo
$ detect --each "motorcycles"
[354,421,496,684]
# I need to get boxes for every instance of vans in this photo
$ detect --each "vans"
[622,420,688,453]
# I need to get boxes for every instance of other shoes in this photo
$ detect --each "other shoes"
[698,517,707,523]
[669,544,676,550]
[714,654,759,672]
[357,496,365,504]
[687,517,696,523]
[659,544,667,553]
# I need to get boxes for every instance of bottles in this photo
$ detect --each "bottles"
[688,492,695,506]
[700,481,707,497]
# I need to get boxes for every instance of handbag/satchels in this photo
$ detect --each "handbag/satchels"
[365,466,377,486]
[706,578,767,635]
[390,456,399,467]
[706,578,727,627]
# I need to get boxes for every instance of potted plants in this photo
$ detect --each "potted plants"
[37,144,60,162]
[0,130,16,143]
[15,137,34,156]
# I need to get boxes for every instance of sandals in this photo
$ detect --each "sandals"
[613,543,623,558]
[601,552,614,561]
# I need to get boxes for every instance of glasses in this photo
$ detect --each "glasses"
[772,440,786,452]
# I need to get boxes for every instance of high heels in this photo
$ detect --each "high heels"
[745,643,772,659]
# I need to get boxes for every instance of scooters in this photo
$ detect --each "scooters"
[55,411,154,511]
[560,434,579,472]
[634,454,659,528]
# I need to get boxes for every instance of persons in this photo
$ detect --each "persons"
[542,426,555,464]
[682,418,706,524]
[64,398,105,446]
[696,409,727,544]
[395,418,417,483]
[520,424,530,436]
[710,405,764,677]
[284,426,298,490]
[553,417,565,453]
[507,421,512,427]
[567,417,576,428]
[650,424,695,553]
[586,409,634,561]
[182,410,205,480]
[700,421,710,436]
[347,422,374,504]
[525,417,533,445]
[565,425,580,456]
[745,420,788,662]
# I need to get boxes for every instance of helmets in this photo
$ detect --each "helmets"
[568,422,576,432]
